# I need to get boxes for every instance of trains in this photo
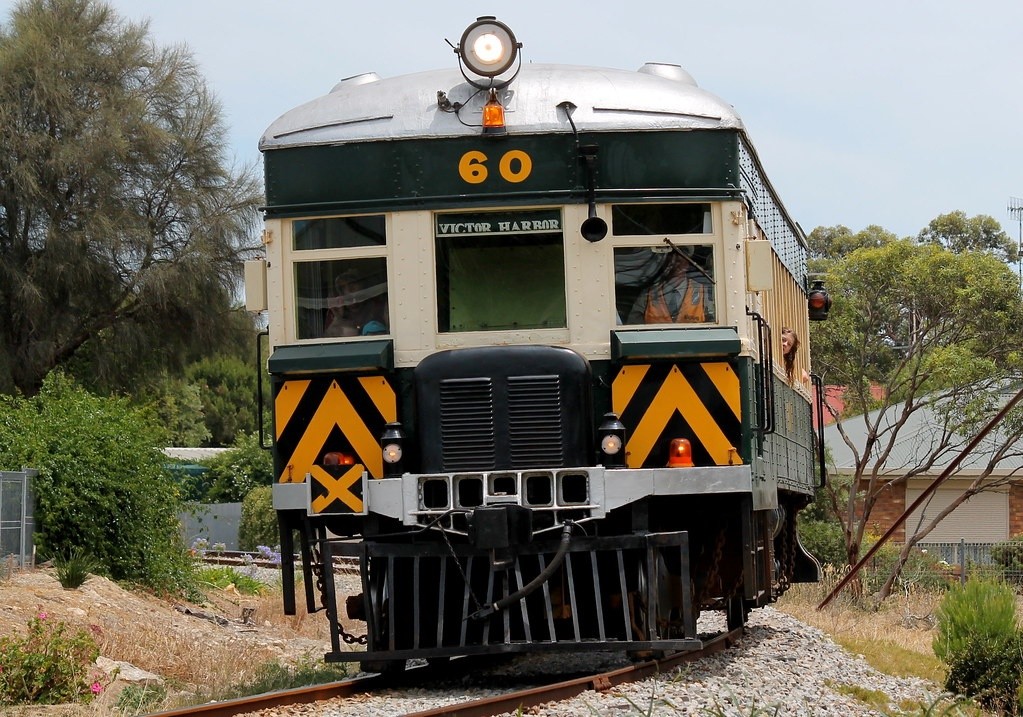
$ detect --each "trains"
[243,16,835,675]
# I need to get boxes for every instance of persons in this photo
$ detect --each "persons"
[323,273,389,336]
[782,327,801,390]
[628,245,705,324]
[801,368,809,384]
